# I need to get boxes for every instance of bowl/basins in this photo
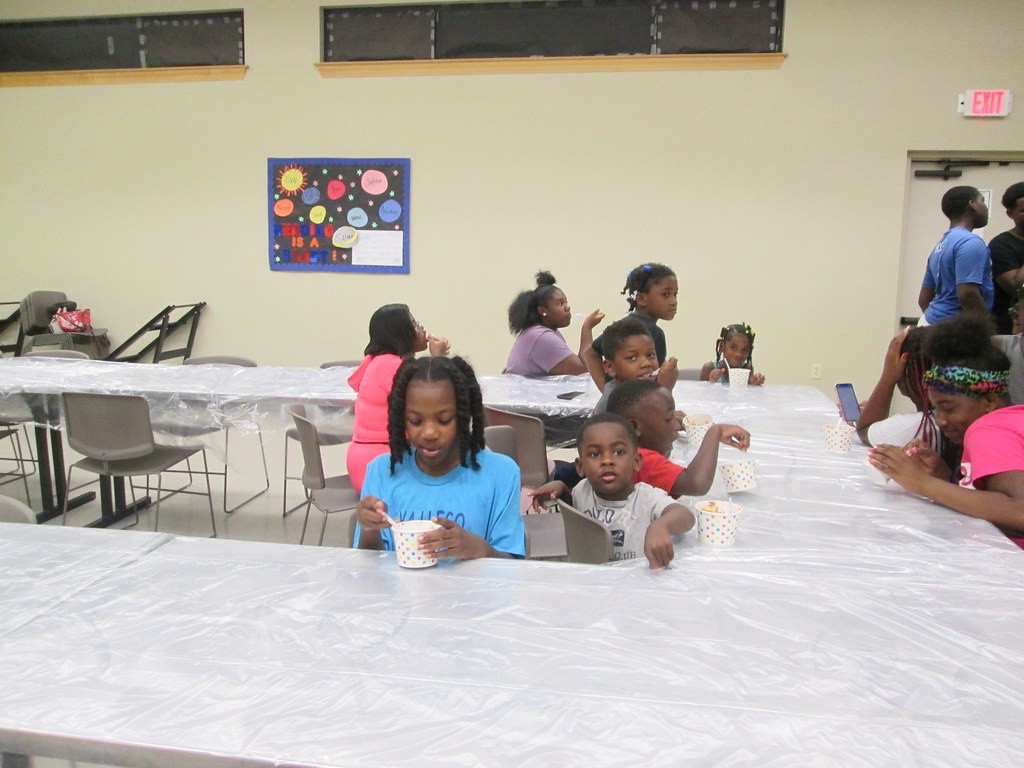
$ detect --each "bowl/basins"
[683,413,715,448]
[728,368,750,387]
[717,457,760,494]
[822,423,856,452]
[390,520,442,568]
[695,500,742,546]
[864,445,892,486]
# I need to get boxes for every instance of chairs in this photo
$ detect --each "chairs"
[0,290,702,566]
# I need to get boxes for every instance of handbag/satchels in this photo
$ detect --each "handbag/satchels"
[51,309,93,332]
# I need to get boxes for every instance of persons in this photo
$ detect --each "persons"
[348,304,451,493]
[606,379,750,496]
[837,181,1024,548]
[352,356,524,559]
[504,271,605,378]
[700,322,766,386]
[592,318,678,415]
[527,412,695,570]
[583,263,678,396]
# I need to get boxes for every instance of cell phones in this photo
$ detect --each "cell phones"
[557,391,584,400]
[835,383,861,421]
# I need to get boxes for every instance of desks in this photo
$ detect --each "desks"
[0,358,1024,768]
